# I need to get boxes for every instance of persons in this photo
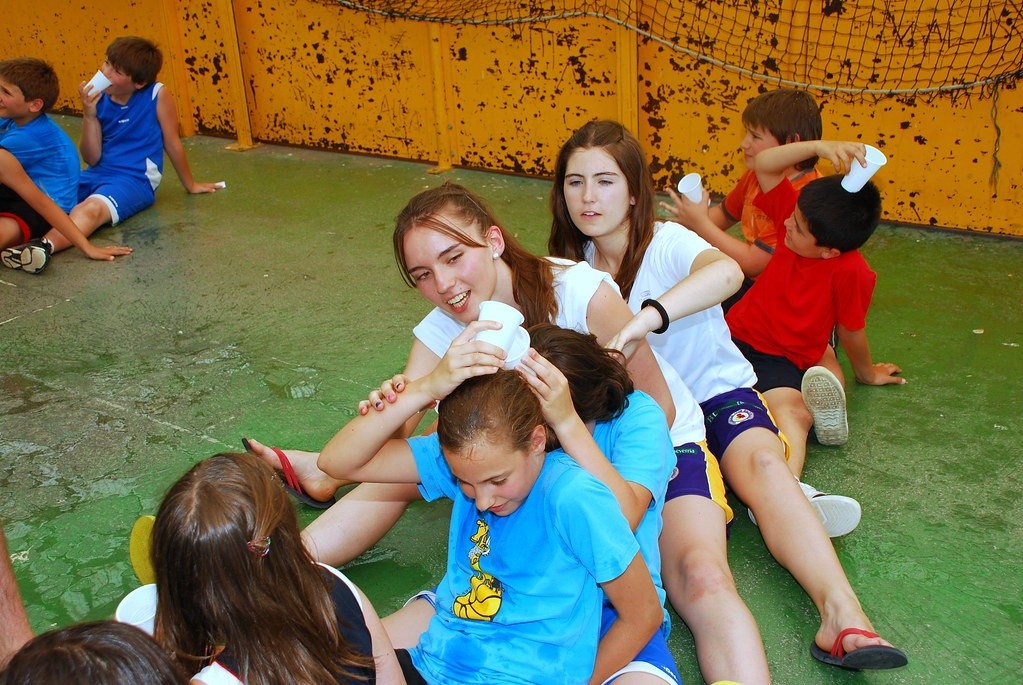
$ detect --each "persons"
[719,139,907,541]
[240,118,910,671]
[0,57,81,248]
[649,87,853,447]
[1,35,223,272]
[150,450,410,685]
[317,322,667,685]
[0,144,134,262]
[368,324,684,685]
[0,523,33,679]
[2,620,195,685]
[291,178,774,685]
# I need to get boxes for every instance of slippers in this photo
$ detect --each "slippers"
[808,629,909,674]
[241,438,335,509]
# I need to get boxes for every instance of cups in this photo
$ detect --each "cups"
[83,70,113,98]
[841,144,887,193]
[115,584,159,637]
[677,171,711,207]
[500,327,532,371]
[473,300,526,356]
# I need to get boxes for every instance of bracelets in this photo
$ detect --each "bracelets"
[641,298,671,335]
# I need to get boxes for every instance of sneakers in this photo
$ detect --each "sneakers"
[1,239,52,273]
[748,483,862,537]
[801,366,849,447]
